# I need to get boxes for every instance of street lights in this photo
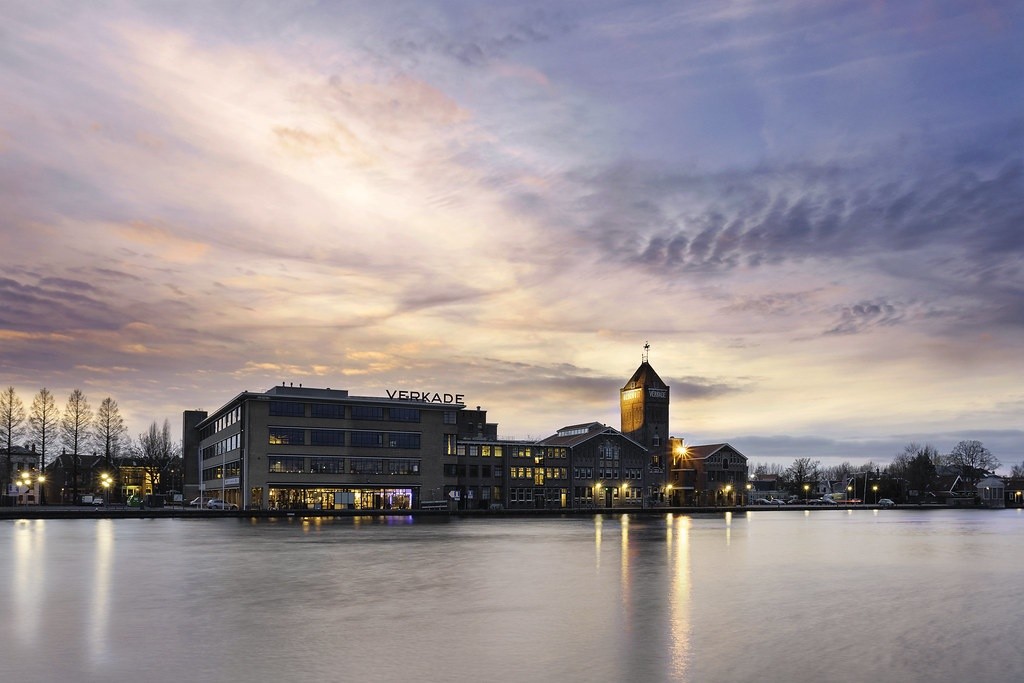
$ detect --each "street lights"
[873,486,878,504]
[38,476,45,506]
[804,485,809,505]
[16,471,31,511]
[1015,492,1021,502]
[101,473,112,507]
[848,486,852,501]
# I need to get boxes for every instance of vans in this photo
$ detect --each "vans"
[190,496,213,508]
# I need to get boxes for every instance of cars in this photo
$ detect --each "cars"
[878,499,894,507]
[755,495,837,505]
[92,498,106,508]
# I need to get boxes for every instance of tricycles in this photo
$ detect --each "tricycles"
[126,495,144,507]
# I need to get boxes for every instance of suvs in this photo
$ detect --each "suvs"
[206,498,239,510]
